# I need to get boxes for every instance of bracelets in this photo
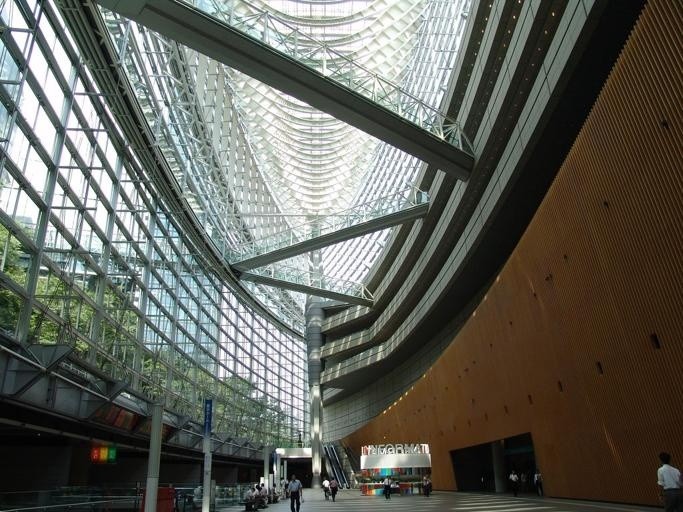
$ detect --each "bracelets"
[657,493,663,496]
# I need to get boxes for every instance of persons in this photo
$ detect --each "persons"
[329,477,339,502]
[287,473,303,512]
[322,479,331,497]
[655,451,683,512]
[422,474,432,497]
[382,475,392,500]
[534,468,544,496]
[520,471,529,492]
[242,483,279,511]
[508,470,520,497]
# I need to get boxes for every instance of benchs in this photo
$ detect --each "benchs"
[266,496,278,504]
[237,501,258,511]
